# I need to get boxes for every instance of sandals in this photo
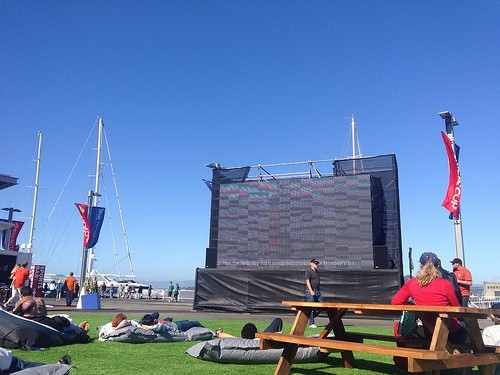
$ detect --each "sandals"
[58,355,71,364]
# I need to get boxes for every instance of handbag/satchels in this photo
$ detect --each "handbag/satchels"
[400,310,417,335]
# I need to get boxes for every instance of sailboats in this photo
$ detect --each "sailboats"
[0,114,166,300]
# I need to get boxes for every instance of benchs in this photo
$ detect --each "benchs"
[255,331,450,360]
[345,330,500,352]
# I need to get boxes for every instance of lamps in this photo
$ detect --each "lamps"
[205,161,225,170]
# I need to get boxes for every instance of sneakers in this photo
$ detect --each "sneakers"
[309,324,317,328]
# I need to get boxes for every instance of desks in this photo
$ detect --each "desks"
[273,301,500,375]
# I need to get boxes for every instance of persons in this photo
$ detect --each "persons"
[391,252,472,345]
[218,317,283,340]
[304,259,324,328]
[65,272,76,306]
[0,261,223,375]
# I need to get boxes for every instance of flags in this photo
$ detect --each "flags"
[74,203,106,250]
[441,130,462,222]
[0,219,26,252]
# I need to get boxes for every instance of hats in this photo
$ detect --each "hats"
[450,258,462,264]
[488,302,500,318]
[311,259,319,264]
[420,252,437,266]
